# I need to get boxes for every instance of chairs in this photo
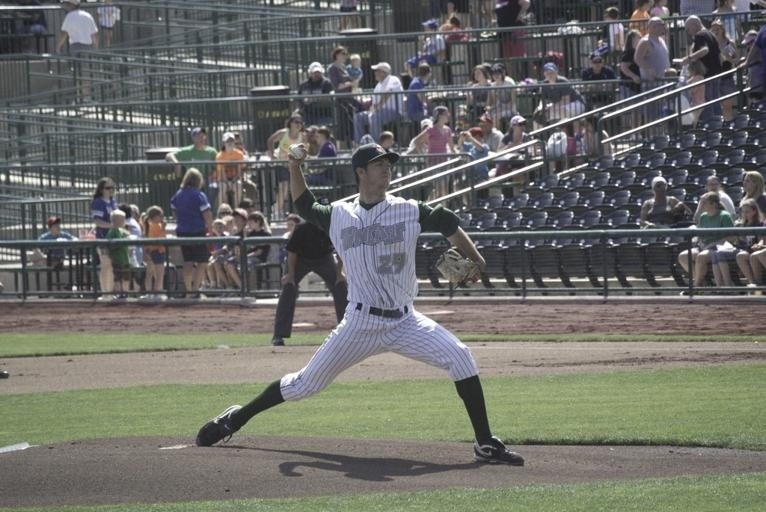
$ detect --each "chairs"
[421,183,698,295]
[534,100,763,205]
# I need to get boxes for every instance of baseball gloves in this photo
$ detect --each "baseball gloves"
[434,245,485,288]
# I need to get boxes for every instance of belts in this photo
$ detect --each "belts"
[355,302,408,318]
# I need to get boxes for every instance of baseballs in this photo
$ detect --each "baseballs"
[287,144,304,160]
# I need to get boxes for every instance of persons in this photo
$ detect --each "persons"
[195,141,526,466]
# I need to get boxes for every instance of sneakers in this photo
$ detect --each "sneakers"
[196,405,242,445]
[271,334,285,346]
[474,436,525,466]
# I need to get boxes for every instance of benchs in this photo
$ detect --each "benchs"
[428,54,568,87]
[258,180,360,223]
[2,260,284,294]
[387,117,415,148]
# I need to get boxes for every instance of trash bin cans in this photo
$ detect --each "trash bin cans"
[249,85,291,152]
[392,0,423,43]
[339,27,379,89]
[144,147,186,217]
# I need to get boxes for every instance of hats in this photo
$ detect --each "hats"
[47,215,61,225]
[510,116,525,127]
[370,61,391,73]
[543,62,557,70]
[351,142,399,167]
